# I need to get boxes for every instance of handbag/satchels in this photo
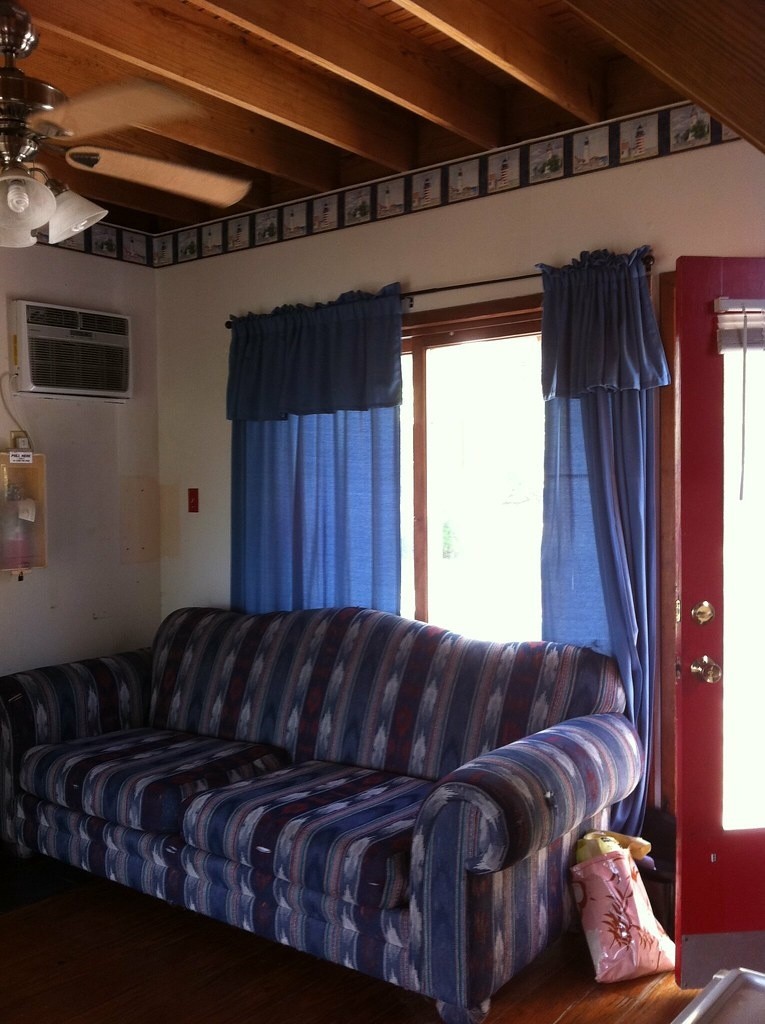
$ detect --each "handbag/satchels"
[570,828,677,984]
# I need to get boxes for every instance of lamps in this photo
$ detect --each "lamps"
[0,74,110,249]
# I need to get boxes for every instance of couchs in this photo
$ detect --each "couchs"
[0,607,645,1024]
